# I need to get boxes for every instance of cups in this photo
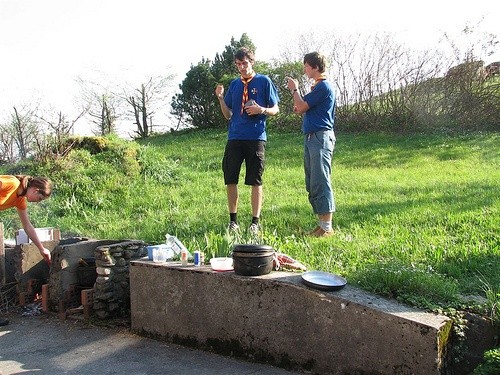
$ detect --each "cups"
[153,249,165,263]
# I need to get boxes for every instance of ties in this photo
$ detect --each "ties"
[310,75,327,90]
[240,71,255,115]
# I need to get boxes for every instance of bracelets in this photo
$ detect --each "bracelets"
[294,89,297,91]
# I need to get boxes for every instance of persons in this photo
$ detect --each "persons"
[0,175,52,264]
[286,52,337,237]
[215,47,280,232]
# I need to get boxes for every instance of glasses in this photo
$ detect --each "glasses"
[235,59,252,67]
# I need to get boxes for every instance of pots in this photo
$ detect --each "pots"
[77,264,97,286]
[230,243,275,276]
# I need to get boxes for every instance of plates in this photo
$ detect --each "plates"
[300,271,347,291]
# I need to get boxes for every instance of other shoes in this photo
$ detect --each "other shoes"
[0,318,9,326]
[305,226,320,237]
[311,228,333,238]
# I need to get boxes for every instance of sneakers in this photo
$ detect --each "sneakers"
[229,221,239,233]
[250,223,259,234]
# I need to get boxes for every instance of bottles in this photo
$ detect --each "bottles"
[194,249,204,267]
[182,249,188,267]
[165,233,192,262]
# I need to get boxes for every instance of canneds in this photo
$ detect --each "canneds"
[182,250,188,267]
[194,251,201,266]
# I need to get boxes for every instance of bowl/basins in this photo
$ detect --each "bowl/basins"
[210,257,233,271]
